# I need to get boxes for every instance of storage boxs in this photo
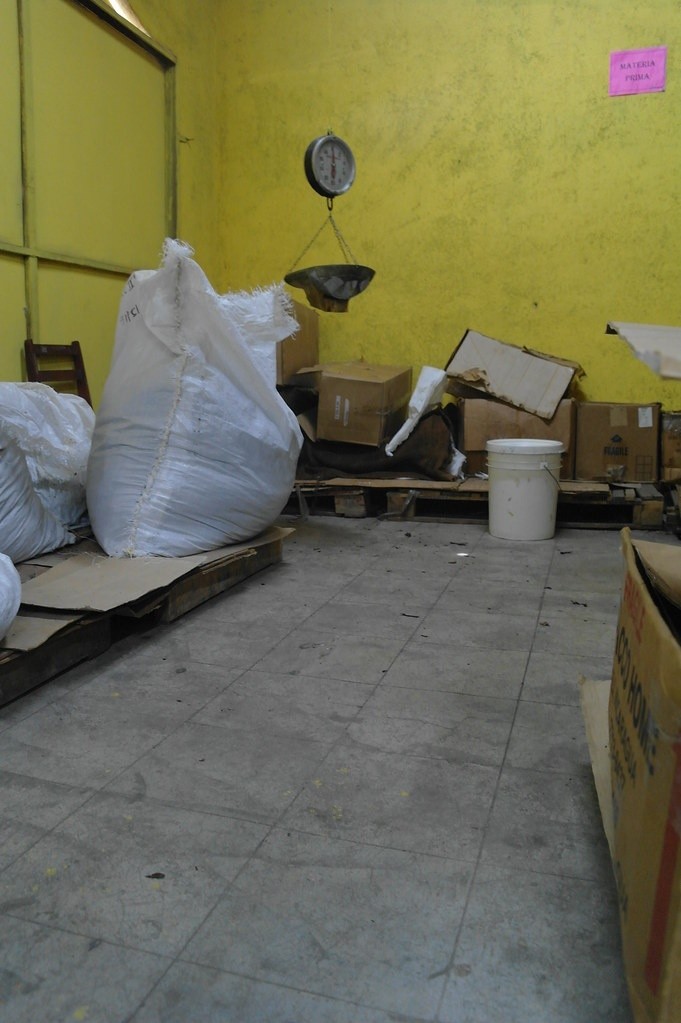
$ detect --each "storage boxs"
[609,527,681,1023]
[445,328,587,421]
[276,299,322,387]
[661,411,681,481]
[457,393,575,481]
[294,359,413,447]
[575,401,661,484]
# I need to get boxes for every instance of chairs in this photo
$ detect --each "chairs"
[25,339,94,409]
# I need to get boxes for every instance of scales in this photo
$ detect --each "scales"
[283,131,377,313]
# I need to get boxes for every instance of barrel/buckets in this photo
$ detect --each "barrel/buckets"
[486,438,564,541]
